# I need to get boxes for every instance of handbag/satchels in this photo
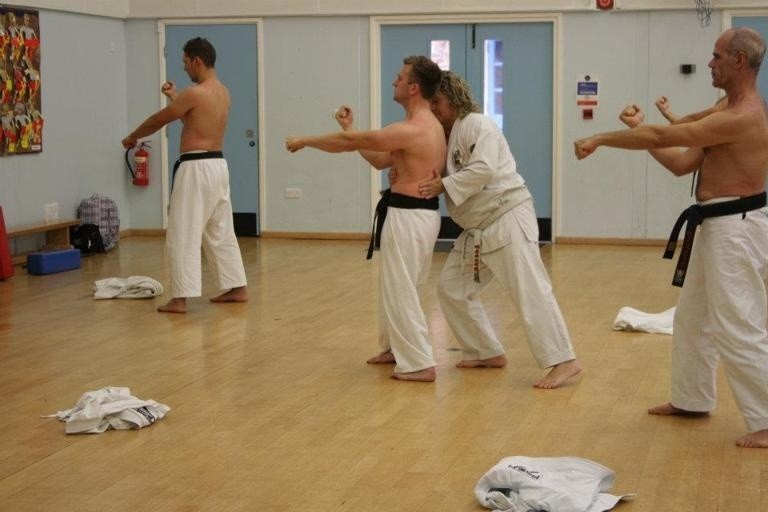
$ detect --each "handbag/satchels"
[77,192,121,250]
[72,222,105,257]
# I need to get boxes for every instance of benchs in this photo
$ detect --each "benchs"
[7,218,83,266]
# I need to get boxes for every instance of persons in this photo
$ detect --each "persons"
[386,72,584,391]
[574,26,768,448]
[652,91,735,127]
[283,52,449,384]
[120,34,251,316]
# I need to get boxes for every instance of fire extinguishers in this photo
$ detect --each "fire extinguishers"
[125,141,152,186]
